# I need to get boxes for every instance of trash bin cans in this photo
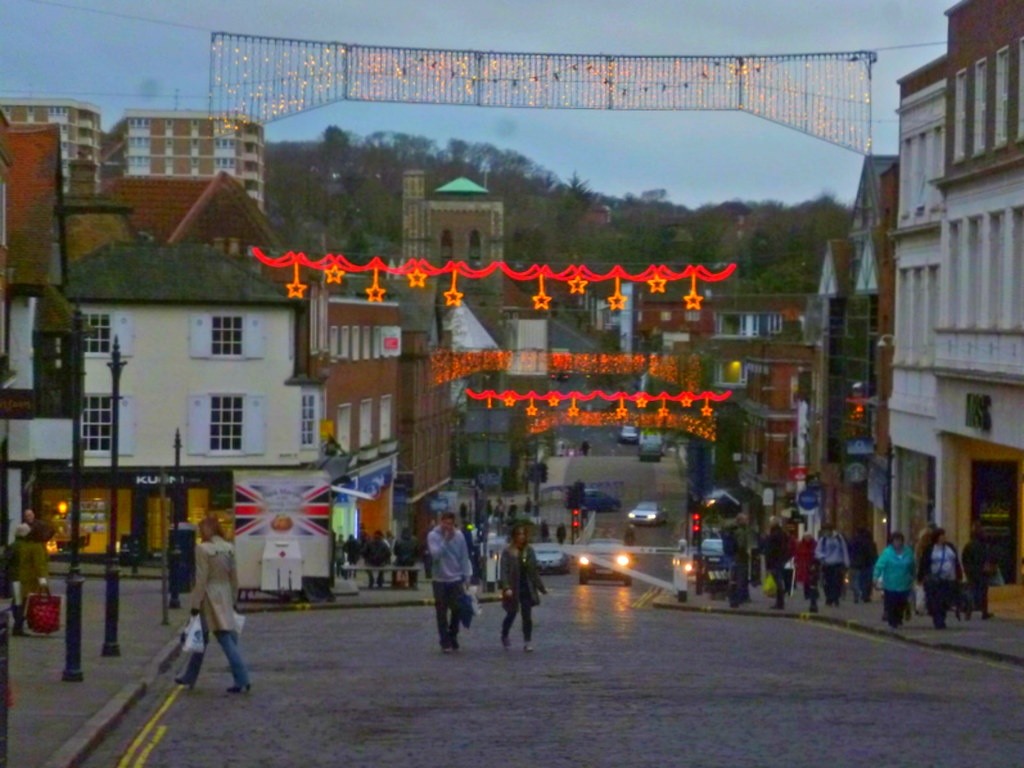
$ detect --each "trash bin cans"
[705,554,727,599]
[170,523,197,592]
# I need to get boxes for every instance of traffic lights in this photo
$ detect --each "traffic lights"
[549,372,570,381]
[542,463,549,483]
[692,512,702,535]
[571,509,579,529]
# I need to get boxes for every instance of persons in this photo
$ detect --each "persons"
[873,531,915,627]
[794,523,851,607]
[726,514,753,607]
[760,515,798,609]
[363,531,392,588]
[962,520,995,620]
[557,523,567,543]
[579,438,591,456]
[462,495,550,652]
[394,527,421,590]
[336,534,346,577]
[175,517,252,693]
[21,507,54,541]
[343,535,362,579]
[359,523,370,543]
[422,525,435,578]
[427,511,473,654]
[913,522,963,629]
[5,524,52,637]
[848,525,878,603]
[624,524,635,541]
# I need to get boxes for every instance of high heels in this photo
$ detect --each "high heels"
[176,677,194,689]
[226,683,251,692]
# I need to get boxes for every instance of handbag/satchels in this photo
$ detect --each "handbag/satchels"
[180,613,204,653]
[921,573,945,593]
[233,610,246,644]
[520,578,540,605]
[762,573,777,597]
[27,587,61,634]
[461,584,483,627]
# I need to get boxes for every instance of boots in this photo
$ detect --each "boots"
[12,605,31,636]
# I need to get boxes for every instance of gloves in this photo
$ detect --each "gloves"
[191,608,199,616]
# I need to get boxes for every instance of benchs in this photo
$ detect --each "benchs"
[338,563,421,591]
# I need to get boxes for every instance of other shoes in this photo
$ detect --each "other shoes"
[441,641,451,651]
[501,635,512,646]
[449,631,460,650]
[981,612,994,619]
[524,644,533,651]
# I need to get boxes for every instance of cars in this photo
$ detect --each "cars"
[532,543,571,576]
[672,538,730,583]
[629,501,670,525]
[565,489,622,513]
[579,539,633,586]
[618,424,667,462]
